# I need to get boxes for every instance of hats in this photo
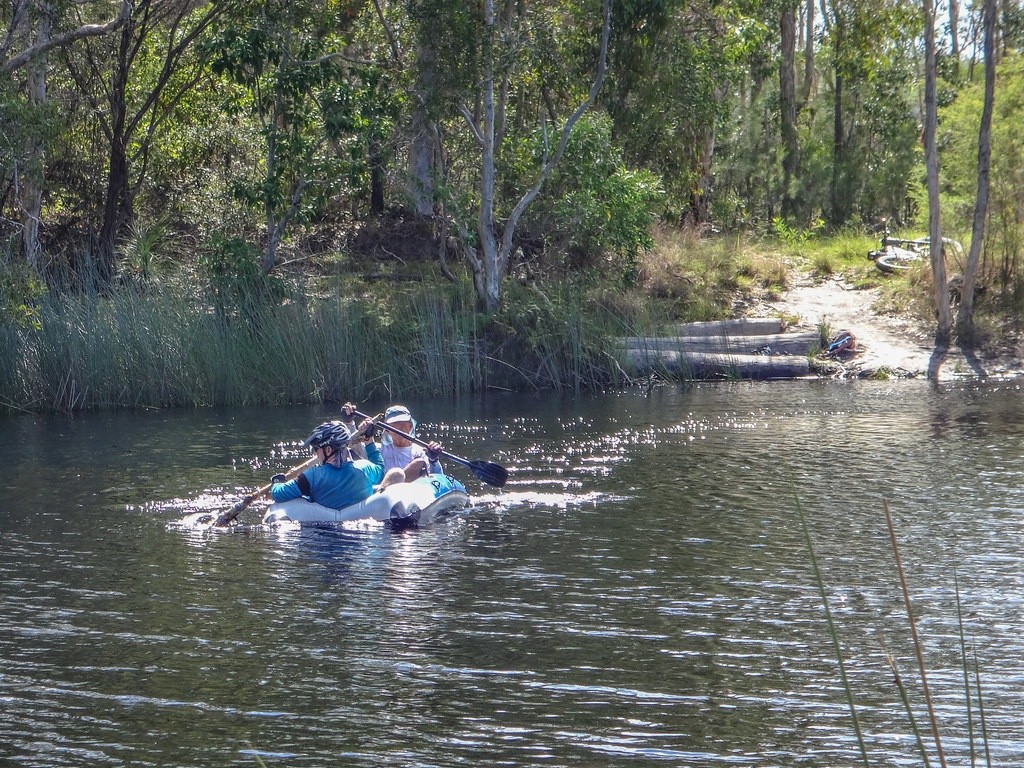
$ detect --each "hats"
[385,405,411,424]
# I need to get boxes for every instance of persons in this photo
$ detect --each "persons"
[271,419,384,510]
[340,401,445,482]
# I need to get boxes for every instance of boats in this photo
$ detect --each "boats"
[262,472,474,530]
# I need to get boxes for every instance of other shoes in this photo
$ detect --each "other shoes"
[380,468,406,491]
[404,456,427,483]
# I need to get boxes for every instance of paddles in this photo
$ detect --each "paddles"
[353,410,509,488]
[214,413,383,527]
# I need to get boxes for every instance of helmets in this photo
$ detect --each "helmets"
[302,421,352,448]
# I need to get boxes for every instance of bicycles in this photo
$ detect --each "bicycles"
[866,215,962,276]
